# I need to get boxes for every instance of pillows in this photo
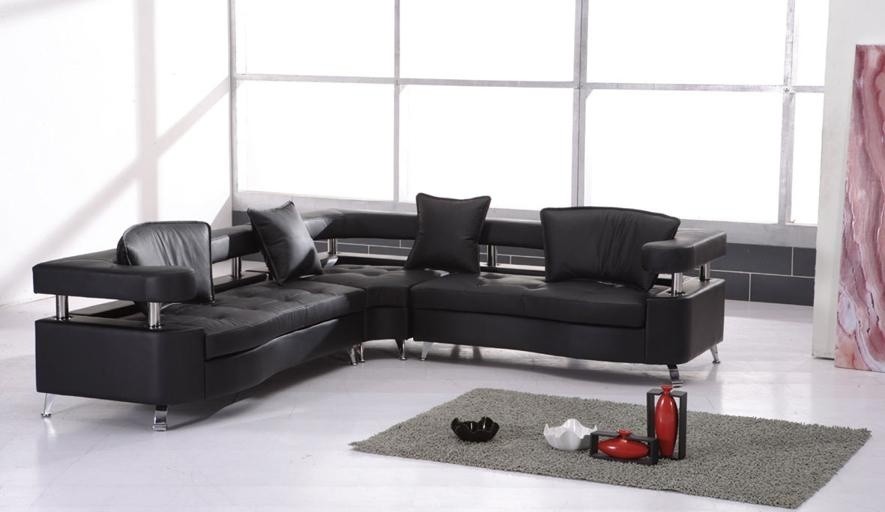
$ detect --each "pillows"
[247,201,324,289]
[404,193,490,274]
[539,207,681,292]
[116,221,214,318]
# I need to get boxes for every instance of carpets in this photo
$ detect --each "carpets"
[347,388,872,509]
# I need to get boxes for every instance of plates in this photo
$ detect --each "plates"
[450,416,498,442]
[542,419,598,452]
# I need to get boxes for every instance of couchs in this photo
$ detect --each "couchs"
[33,207,726,432]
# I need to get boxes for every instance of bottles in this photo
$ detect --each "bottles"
[597,384,679,461]
